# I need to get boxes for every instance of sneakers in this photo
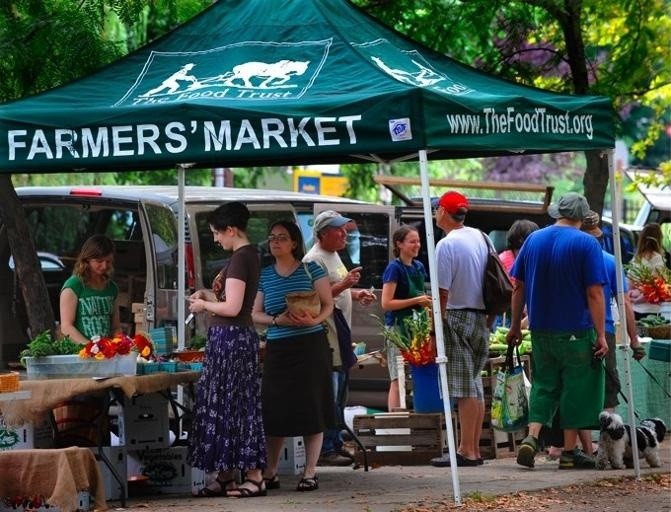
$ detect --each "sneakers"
[545,442,599,471]
[516,435,540,469]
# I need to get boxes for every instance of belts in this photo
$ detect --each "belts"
[446,308,487,315]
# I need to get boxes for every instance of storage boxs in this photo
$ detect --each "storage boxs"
[137,445,208,496]
[0,403,127,511]
[112,387,170,450]
[264,434,307,476]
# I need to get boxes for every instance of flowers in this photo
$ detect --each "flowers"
[369,307,444,366]
[79,331,156,361]
[624,259,671,305]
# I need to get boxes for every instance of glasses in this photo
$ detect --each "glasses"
[267,234,290,242]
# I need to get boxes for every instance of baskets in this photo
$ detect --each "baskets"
[137,327,207,377]
[639,324,671,340]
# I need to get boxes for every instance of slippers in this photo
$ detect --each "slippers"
[429,450,484,469]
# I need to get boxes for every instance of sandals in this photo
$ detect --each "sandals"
[263,473,280,489]
[297,476,319,492]
[191,477,237,499]
[226,478,268,499]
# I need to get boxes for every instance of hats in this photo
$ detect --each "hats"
[313,210,353,235]
[579,209,604,238]
[547,191,591,222]
[431,191,470,217]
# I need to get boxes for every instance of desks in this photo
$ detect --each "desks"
[1,359,371,510]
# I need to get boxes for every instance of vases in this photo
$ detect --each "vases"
[411,362,444,413]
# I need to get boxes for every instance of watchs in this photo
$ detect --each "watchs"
[273,316,278,327]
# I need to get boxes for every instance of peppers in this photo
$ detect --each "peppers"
[635,315,670,328]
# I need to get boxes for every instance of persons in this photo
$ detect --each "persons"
[494,219,544,332]
[186,200,271,498]
[629,236,671,282]
[547,415,598,457]
[631,222,671,271]
[580,206,647,456]
[508,192,611,470]
[381,226,434,410]
[59,235,122,346]
[252,218,337,490]
[302,210,377,467]
[431,190,500,465]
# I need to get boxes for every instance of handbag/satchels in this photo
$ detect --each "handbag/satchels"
[321,305,358,371]
[478,228,514,317]
[490,337,532,432]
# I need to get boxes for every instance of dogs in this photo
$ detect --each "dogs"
[594,411,666,470]
